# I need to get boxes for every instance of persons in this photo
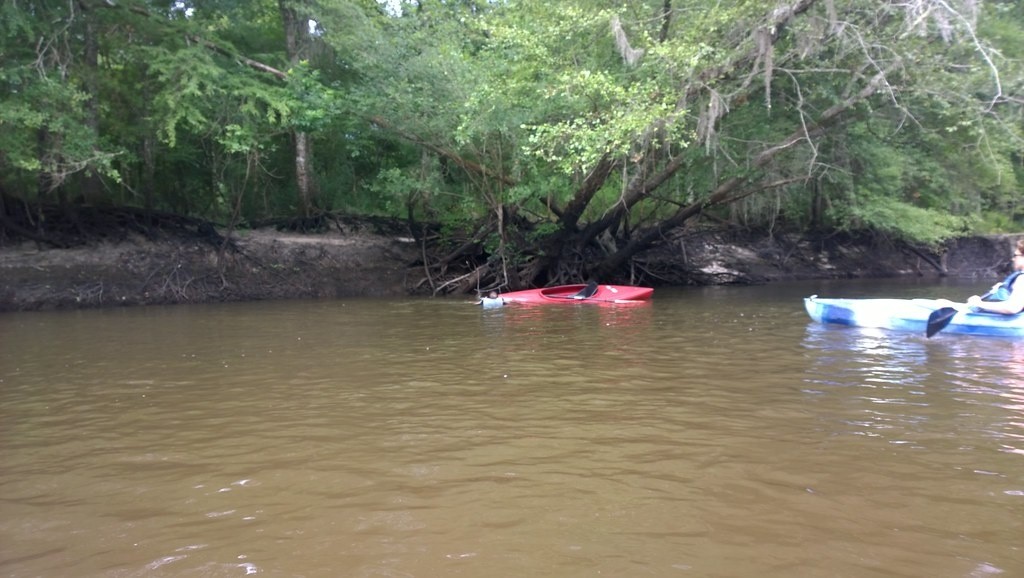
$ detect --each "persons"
[475,291,509,309]
[968,240,1024,314]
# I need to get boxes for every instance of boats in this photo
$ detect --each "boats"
[484,282,655,313]
[802,290,1024,343]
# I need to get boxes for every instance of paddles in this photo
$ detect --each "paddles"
[924,283,1004,340]
[545,294,645,304]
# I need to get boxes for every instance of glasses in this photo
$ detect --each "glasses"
[1013,254,1024,257]
